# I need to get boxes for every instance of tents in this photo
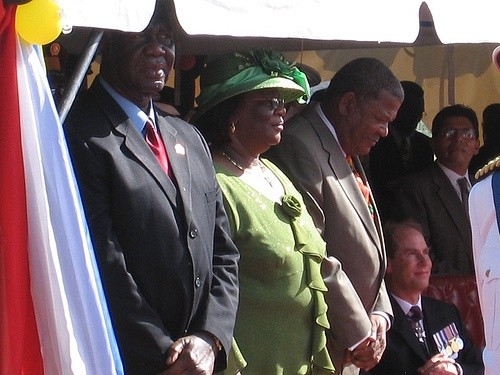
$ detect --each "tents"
[60,0,500,125]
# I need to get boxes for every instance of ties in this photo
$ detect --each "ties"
[410,306,430,357]
[457,177,469,217]
[142,118,177,189]
[346,154,374,222]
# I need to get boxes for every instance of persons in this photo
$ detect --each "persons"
[62,4,241,375]
[360,45,500,375]
[194,48,335,375]
[263,57,406,375]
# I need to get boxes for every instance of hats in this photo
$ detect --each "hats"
[189,42,310,125]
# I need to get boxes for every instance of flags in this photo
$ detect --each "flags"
[0,0,125,375]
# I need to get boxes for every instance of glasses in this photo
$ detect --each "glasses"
[249,97,285,109]
[439,127,476,141]
[50,88,66,96]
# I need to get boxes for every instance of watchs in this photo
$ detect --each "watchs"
[213,334,223,352]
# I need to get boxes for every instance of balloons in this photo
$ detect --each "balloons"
[15,0,63,45]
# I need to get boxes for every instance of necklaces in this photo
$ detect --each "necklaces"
[219,146,252,176]
